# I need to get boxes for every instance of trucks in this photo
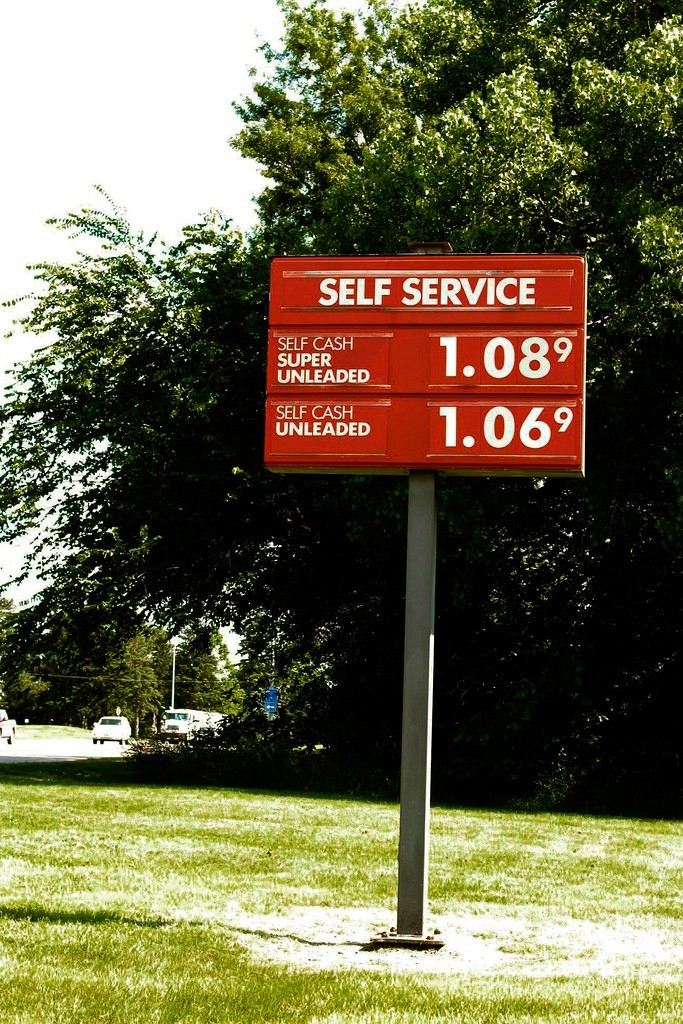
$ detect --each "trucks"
[161,708,222,741]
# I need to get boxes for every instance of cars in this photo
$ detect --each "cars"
[91,716,131,745]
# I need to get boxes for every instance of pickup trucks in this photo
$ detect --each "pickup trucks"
[0,709,16,743]
